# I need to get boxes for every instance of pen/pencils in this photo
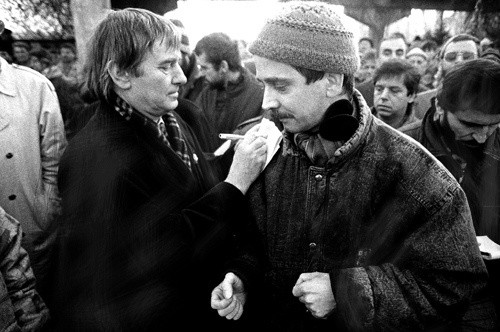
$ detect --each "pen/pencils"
[219,133,244,139]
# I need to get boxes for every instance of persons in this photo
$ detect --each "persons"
[397,59,500,261]
[57,8,267,332]
[0,206,52,332]
[211,0,490,332]
[0,32,500,148]
[0,19,69,312]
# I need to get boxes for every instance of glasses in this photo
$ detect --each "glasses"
[443,51,478,62]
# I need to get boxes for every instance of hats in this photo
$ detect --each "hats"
[480,38,493,46]
[59,35,76,54]
[406,47,427,60]
[247,2,362,76]
[480,43,500,58]
